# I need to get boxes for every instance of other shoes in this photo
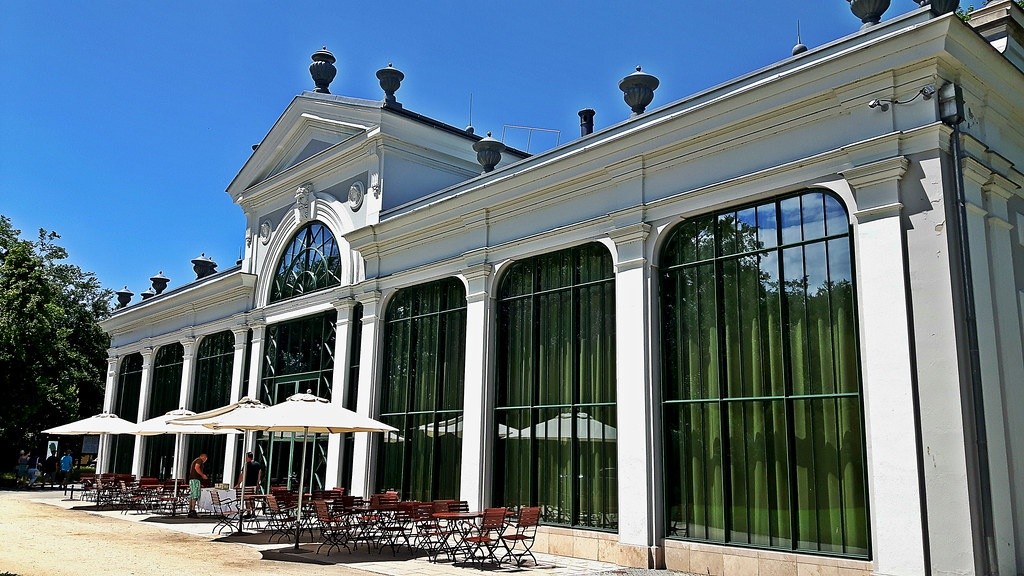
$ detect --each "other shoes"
[243,513,250,518]
[188,510,200,519]
[250,514,255,518]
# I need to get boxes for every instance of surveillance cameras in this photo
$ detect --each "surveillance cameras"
[869,99,879,108]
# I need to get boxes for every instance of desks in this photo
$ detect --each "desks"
[432,512,481,565]
[356,505,411,557]
[197,488,238,514]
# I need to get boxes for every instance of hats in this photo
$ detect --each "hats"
[67,449,73,454]
[20,450,25,454]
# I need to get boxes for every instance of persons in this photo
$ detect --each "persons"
[16,449,75,494]
[233,451,261,518]
[188,453,208,519]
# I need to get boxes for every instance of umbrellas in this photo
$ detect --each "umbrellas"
[419,418,517,440]
[135,406,239,518]
[511,413,621,445]
[40,411,135,510]
[205,392,398,553]
[167,395,278,533]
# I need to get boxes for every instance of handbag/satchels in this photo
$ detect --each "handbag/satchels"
[18,464,29,473]
[34,470,41,477]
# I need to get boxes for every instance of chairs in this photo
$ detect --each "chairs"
[78,472,204,520]
[210,486,542,571]
